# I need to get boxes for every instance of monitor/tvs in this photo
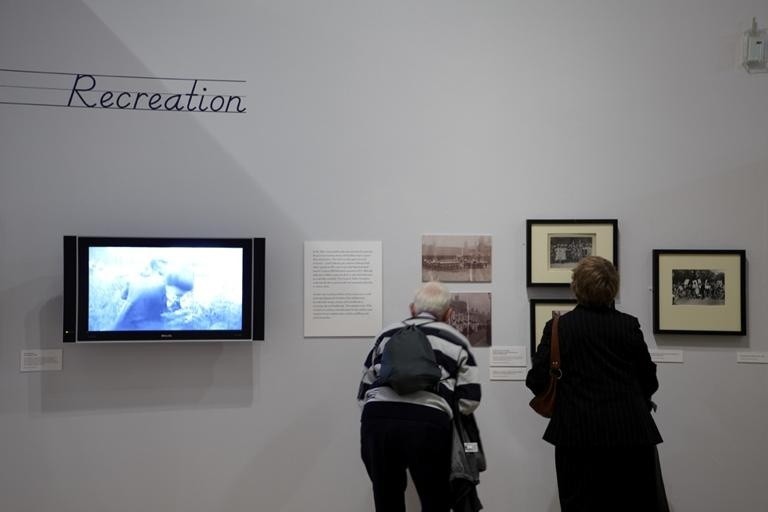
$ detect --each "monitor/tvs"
[61,234,267,341]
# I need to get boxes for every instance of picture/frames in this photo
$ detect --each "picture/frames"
[653,249,746,335]
[530,298,615,358]
[527,219,618,288]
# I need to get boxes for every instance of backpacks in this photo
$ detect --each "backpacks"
[378,322,443,397]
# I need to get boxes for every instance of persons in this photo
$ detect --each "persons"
[114,272,194,330]
[357,282,483,512]
[672,276,723,300]
[525,255,669,512]
[551,240,592,264]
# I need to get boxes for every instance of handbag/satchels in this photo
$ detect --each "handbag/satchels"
[527,309,561,419]
